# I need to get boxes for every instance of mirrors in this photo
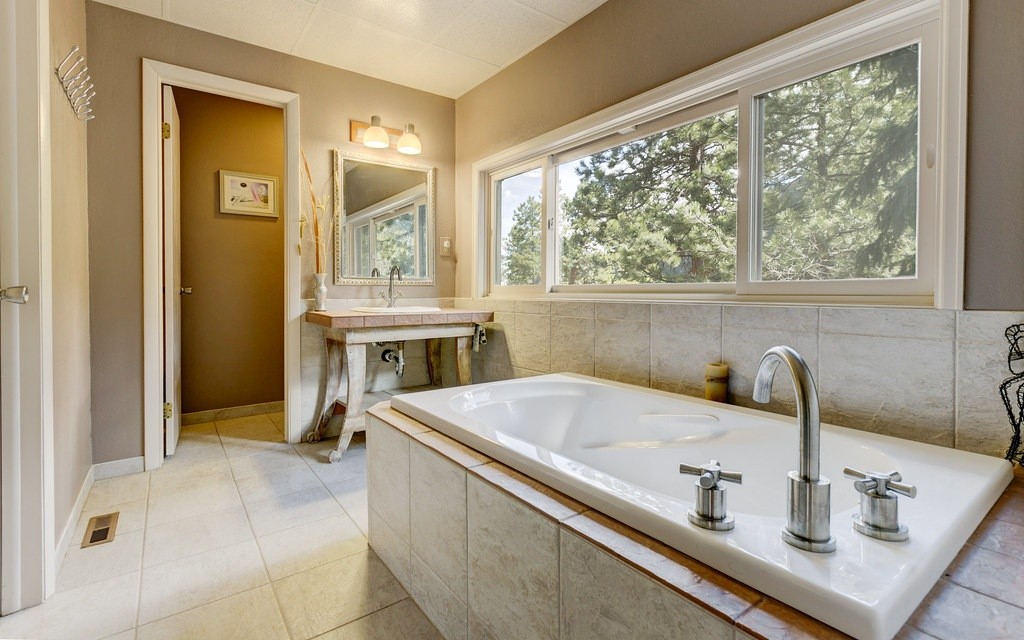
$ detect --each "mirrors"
[333,148,437,286]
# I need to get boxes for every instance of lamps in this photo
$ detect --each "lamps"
[357,115,421,155]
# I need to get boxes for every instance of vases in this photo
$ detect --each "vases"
[312,273,327,311]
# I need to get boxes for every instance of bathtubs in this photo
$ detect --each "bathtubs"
[390,371,1012,611]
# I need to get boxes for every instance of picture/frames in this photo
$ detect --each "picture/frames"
[219,169,279,218]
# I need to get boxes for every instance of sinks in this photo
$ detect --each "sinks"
[354,306,441,312]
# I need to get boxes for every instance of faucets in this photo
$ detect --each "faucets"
[751,345,839,554]
[377,265,405,309]
[371,268,381,277]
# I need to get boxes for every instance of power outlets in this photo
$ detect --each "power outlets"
[439,237,451,256]
